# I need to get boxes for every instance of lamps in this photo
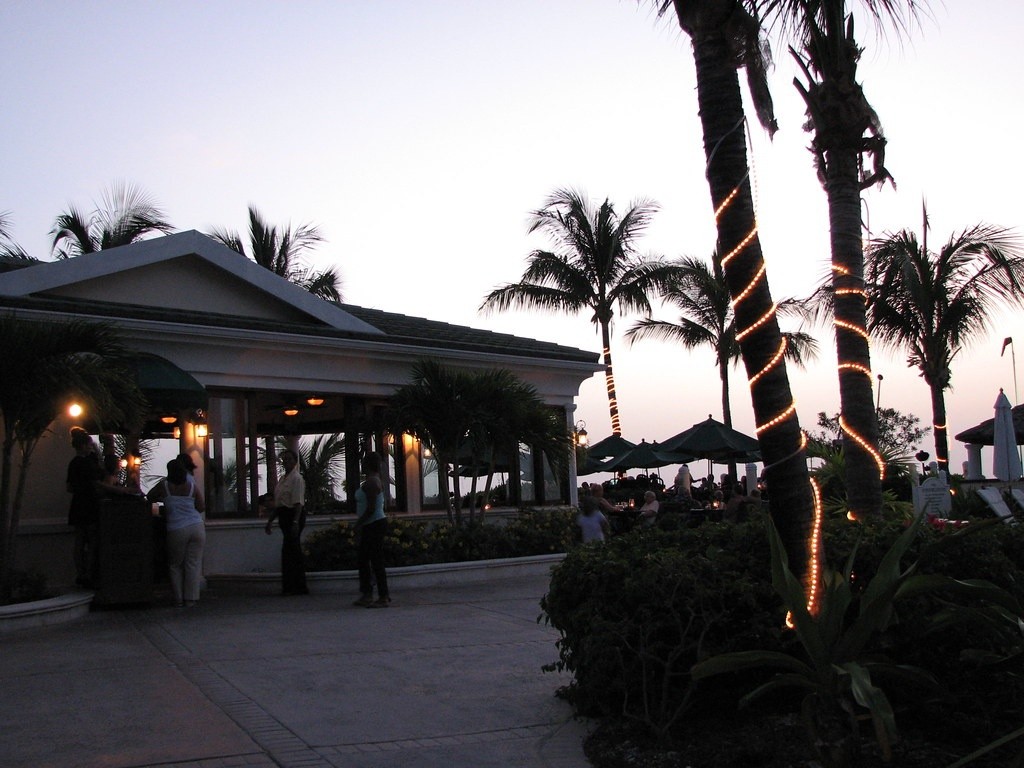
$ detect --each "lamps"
[173,426,180,439]
[161,417,177,423]
[574,420,587,446]
[197,419,208,437]
[388,434,394,444]
[121,453,128,467]
[286,398,325,417]
[132,452,142,464]
[423,447,432,459]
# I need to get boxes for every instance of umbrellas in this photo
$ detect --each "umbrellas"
[994,393,1021,515]
[449,417,764,484]
[955,404,1024,445]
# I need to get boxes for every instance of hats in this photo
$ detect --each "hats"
[177,453,198,470]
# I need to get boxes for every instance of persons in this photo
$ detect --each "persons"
[577,465,768,542]
[352,452,392,608]
[266,450,309,595]
[66,426,206,612]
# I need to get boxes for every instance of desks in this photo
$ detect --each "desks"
[610,510,647,538]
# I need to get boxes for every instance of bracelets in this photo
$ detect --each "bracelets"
[292,521,298,523]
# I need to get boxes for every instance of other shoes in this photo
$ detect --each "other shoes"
[185,602,200,609]
[359,597,373,605]
[382,598,391,607]
[168,603,185,610]
